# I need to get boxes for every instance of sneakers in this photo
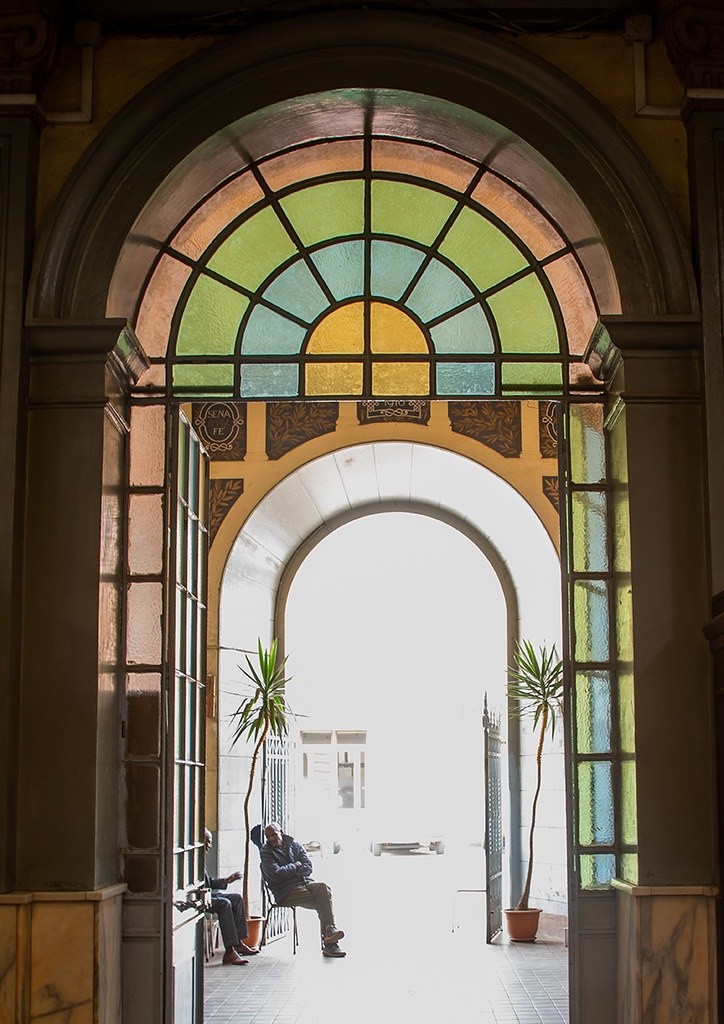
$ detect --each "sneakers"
[321,925,346,957]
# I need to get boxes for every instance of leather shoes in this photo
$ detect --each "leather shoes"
[223,950,250,964]
[234,940,260,955]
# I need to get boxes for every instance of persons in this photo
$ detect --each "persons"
[260,823,346,957]
[204,826,259,965]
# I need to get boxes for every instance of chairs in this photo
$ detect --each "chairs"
[205,911,220,963]
[259,862,301,955]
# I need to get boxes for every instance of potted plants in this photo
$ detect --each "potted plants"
[227,637,310,949]
[502,637,564,941]
